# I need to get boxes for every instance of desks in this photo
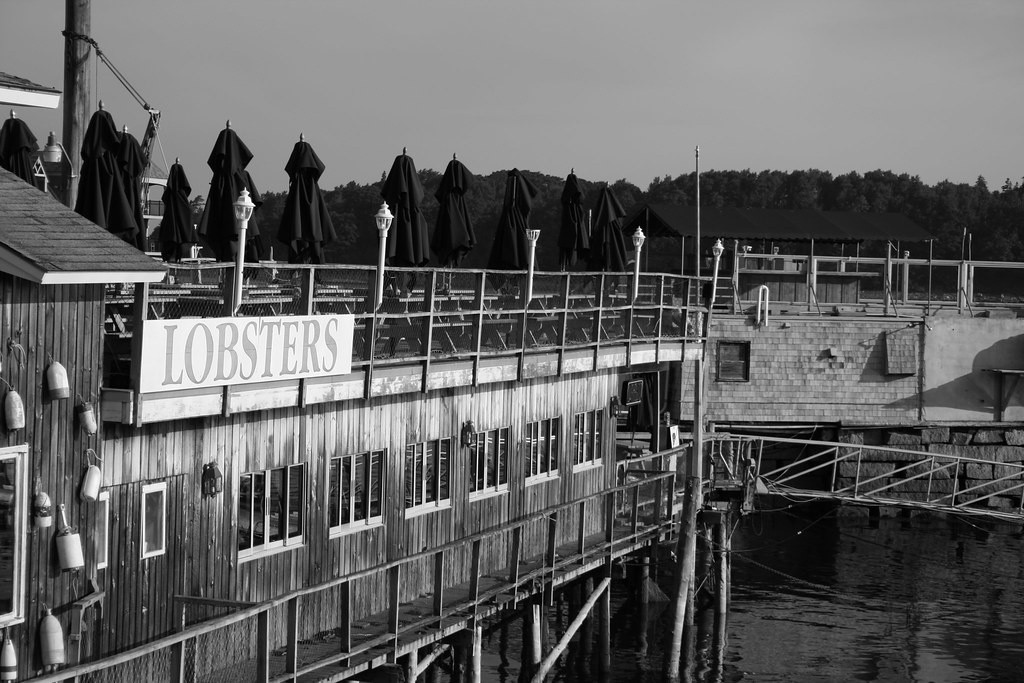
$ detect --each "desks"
[239,508,298,543]
[105,284,674,345]
[981,366,1024,423]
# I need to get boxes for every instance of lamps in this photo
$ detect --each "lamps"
[203,460,222,497]
[461,419,475,445]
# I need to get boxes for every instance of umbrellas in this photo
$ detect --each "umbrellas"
[557,168,628,291]
[74,100,149,295]
[158,120,265,284]
[377,148,478,298]
[0,109,40,189]
[276,133,337,283]
[628,374,654,469]
[487,168,540,291]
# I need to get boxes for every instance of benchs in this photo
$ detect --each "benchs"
[354,314,657,360]
